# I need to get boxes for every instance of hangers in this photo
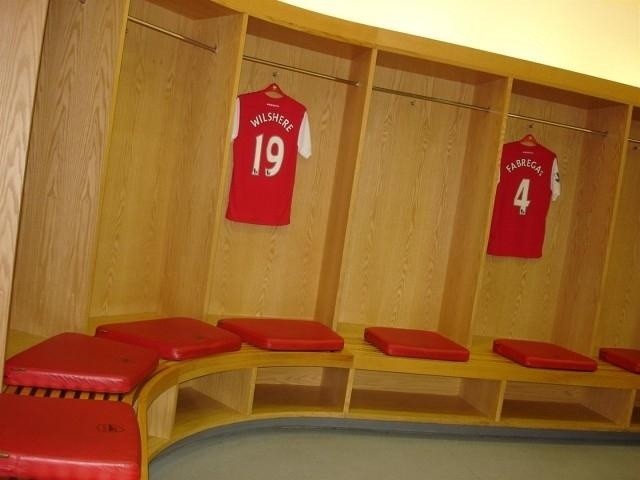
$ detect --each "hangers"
[263,59,284,97]
[520,117,540,145]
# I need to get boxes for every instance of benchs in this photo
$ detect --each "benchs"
[0,308,640,480]
